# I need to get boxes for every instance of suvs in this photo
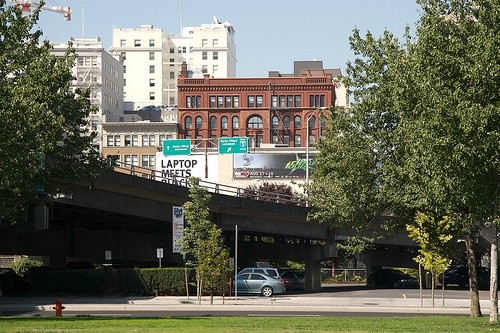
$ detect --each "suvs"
[235,268,281,279]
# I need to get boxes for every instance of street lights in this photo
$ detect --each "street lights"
[305,107,329,203]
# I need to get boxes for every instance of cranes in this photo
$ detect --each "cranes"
[11,0,72,23]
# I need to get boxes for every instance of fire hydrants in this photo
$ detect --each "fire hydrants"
[53,297,65,316]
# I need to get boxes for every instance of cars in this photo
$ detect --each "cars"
[438,266,490,290]
[232,273,287,297]
[368,269,418,289]
